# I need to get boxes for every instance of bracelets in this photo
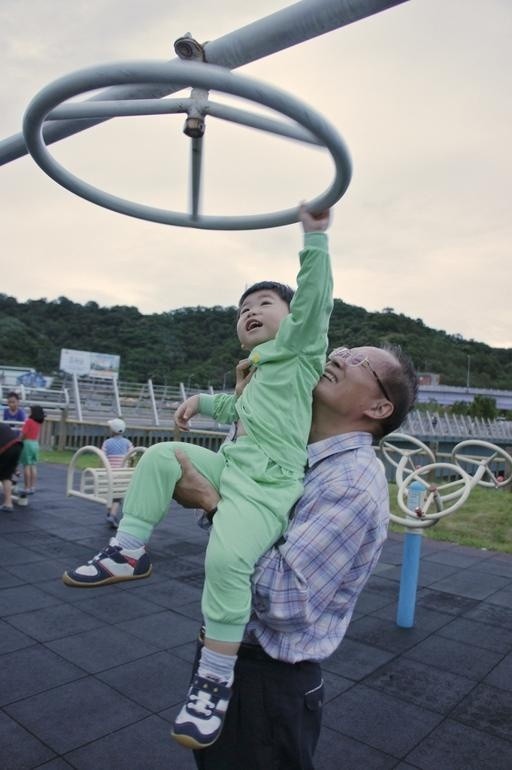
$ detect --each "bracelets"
[205,506,218,523]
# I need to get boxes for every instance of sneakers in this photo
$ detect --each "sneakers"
[171,669,234,751]
[0,468,35,511]
[62,536,153,588]
[106,513,119,528]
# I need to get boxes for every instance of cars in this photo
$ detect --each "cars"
[68,390,181,413]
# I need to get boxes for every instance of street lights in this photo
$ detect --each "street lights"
[464,352,471,389]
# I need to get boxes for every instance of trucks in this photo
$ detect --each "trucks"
[0,365,59,402]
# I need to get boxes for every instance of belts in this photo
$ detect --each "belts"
[198,625,266,658]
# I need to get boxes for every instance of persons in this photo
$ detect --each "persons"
[100,417,136,528]
[63,197,334,749]
[0,421,26,511]
[3,390,26,478]
[175,339,418,769]
[18,405,46,494]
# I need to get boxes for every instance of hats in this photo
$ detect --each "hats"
[108,418,126,434]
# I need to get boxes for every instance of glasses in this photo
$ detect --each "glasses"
[328,346,396,421]
